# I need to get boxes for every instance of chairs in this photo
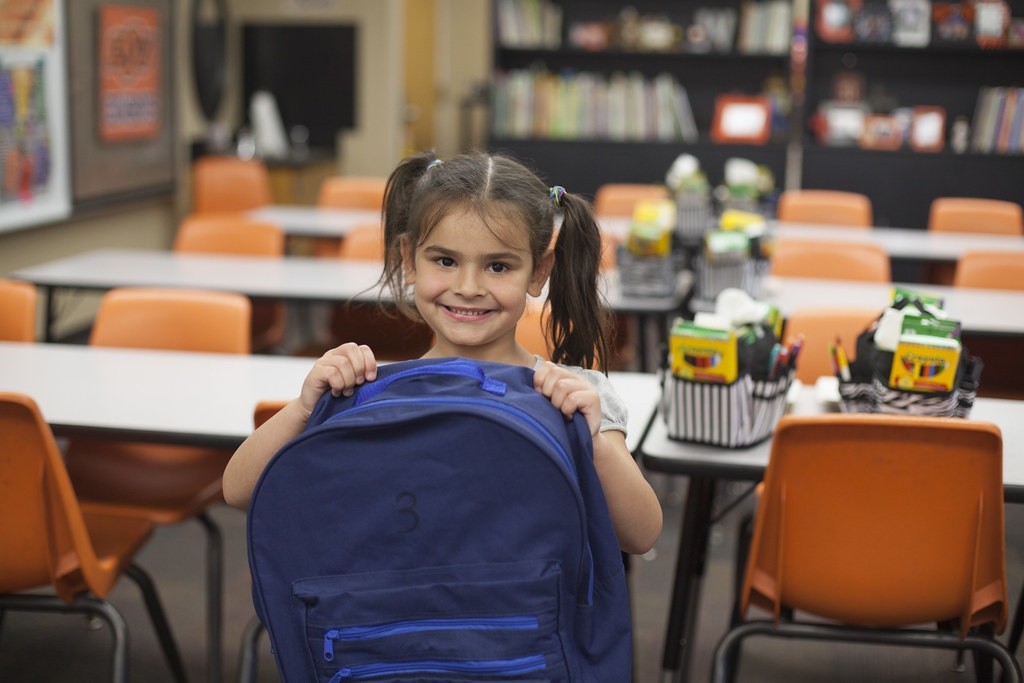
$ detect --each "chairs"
[0,155,1024,683]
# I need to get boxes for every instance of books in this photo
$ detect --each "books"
[495,0,1024,152]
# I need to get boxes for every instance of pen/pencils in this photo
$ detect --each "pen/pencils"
[769,307,806,380]
[829,337,852,382]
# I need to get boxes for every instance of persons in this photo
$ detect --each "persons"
[221,150,664,555]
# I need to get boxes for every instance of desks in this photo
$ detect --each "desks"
[638,382,1024,683]
[762,222,1024,263]
[12,251,698,376]
[688,275,1023,342]
[0,341,665,577]
[252,206,633,243]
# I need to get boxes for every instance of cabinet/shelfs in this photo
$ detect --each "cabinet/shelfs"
[488,0,1024,284]
